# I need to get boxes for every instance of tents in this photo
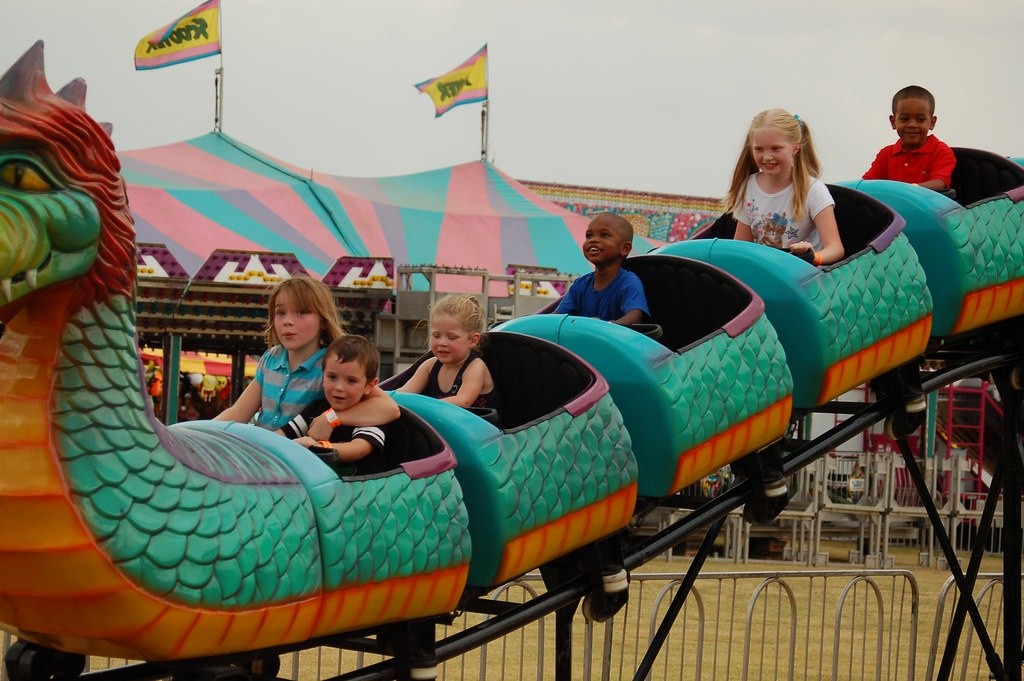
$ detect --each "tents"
[114,130,673,294]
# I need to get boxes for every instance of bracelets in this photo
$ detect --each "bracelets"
[324,407,341,428]
[815,253,823,265]
[318,440,331,448]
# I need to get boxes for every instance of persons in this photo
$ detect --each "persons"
[719,108,845,266]
[550,211,651,328]
[271,334,391,475]
[209,273,401,441]
[394,293,504,427]
[861,84,956,190]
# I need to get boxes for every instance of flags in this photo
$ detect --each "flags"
[134,0,222,69]
[413,42,487,117]
